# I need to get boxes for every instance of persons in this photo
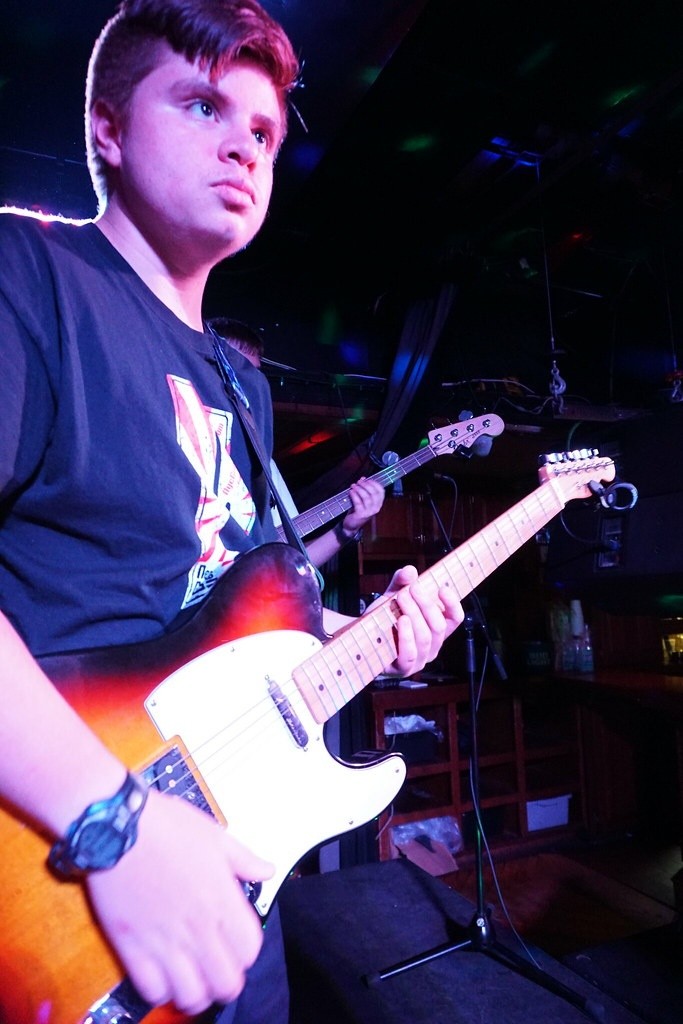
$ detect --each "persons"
[211,318,386,570]
[0,0,466,1024]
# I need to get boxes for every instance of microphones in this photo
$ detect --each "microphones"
[381,451,451,482]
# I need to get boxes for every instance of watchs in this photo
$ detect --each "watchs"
[334,521,364,546]
[48,771,151,881]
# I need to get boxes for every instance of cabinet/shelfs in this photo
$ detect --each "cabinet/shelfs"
[356,488,587,863]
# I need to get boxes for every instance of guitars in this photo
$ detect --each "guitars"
[0,447,638,1024]
[270,409,505,546]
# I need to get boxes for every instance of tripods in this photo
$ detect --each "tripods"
[362,483,591,1009]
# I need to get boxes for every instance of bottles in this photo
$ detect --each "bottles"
[554,615,576,671]
[570,599,584,669]
[578,624,595,673]
